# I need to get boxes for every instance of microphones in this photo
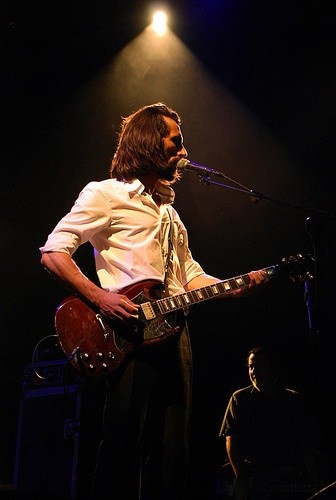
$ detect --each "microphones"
[177,158,224,177]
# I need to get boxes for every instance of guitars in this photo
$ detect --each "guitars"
[54,248,315,377]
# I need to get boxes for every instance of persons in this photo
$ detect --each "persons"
[39,103,267,500]
[220,347,306,500]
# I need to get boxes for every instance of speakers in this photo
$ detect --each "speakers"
[13,383,108,500]
